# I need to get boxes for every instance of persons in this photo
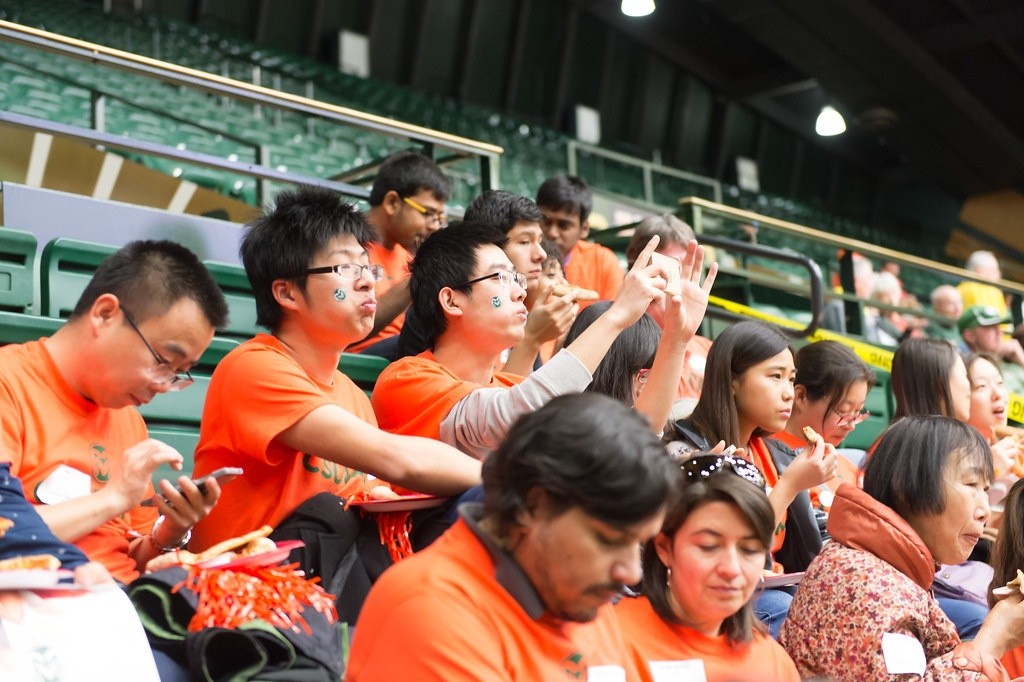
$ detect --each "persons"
[536,173,625,314]
[343,151,453,361]
[613,454,801,682]
[987,478,1024,682]
[564,300,745,458]
[766,340,876,545]
[489,245,570,311]
[961,349,1024,480]
[188,183,484,627]
[0,239,229,682]
[344,392,686,682]
[856,336,1024,564]
[779,416,1024,682]
[463,189,579,391]
[828,248,1024,393]
[626,210,714,422]
[1,464,162,681]
[660,317,839,641]
[370,219,719,513]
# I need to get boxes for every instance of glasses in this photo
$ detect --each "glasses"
[118,302,193,392]
[401,198,447,230]
[281,260,383,282]
[821,395,873,424]
[681,454,767,491]
[450,268,529,290]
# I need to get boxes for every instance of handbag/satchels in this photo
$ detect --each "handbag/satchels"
[929,560,995,606]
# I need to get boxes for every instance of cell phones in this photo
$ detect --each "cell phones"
[650,251,682,297]
[141,466,244,506]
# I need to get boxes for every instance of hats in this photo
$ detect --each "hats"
[957,304,1013,334]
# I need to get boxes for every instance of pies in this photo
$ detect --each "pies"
[1007,569,1024,599]
[803,426,820,444]
[994,425,1024,477]
[146,526,274,567]
[538,276,599,300]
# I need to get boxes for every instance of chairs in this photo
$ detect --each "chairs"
[1,0,1024,498]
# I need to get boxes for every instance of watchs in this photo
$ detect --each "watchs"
[149,514,192,554]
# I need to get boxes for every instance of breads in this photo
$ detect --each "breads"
[0,554,61,571]
[243,538,276,556]
[371,485,398,499]
